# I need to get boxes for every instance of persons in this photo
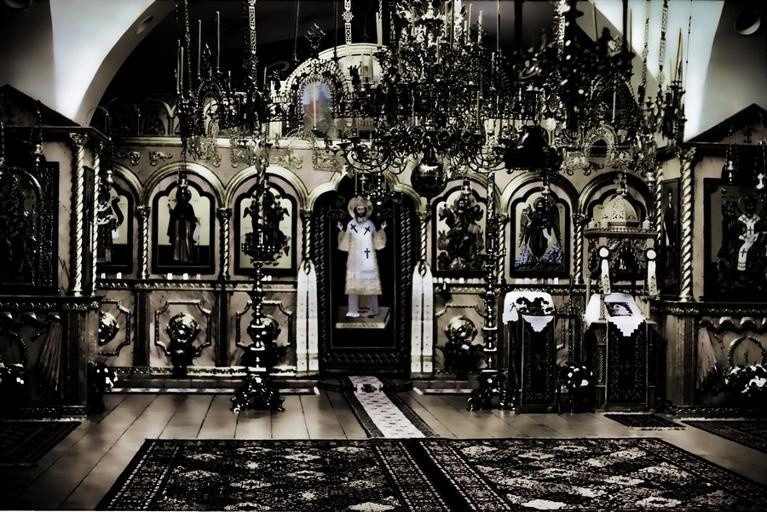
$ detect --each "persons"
[166,189,199,264]
[445,197,479,261]
[527,201,552,262]
[337,205,387,317]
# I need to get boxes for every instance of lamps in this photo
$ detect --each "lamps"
[171,5,693,225]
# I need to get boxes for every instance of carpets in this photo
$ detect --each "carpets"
[97,436,766,510]
